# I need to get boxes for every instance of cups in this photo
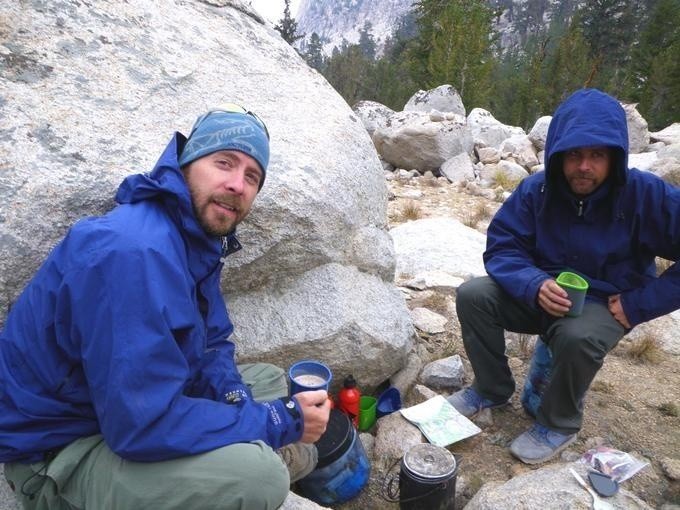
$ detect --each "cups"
[556,270,589,317]
[287,359,334,400]
[356,387,401,430]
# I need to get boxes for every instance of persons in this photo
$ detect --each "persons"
[1,108,332,509]
[444,86,680,463]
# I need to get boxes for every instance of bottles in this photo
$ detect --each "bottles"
[338,373,361,430]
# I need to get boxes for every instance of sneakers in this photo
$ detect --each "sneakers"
[445,385,509,421]
[508,419,577,465]
[275,442,319,485]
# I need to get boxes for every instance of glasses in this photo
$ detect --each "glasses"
[182,103,268,157]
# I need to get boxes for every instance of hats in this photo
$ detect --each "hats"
[178,110,270,194]
